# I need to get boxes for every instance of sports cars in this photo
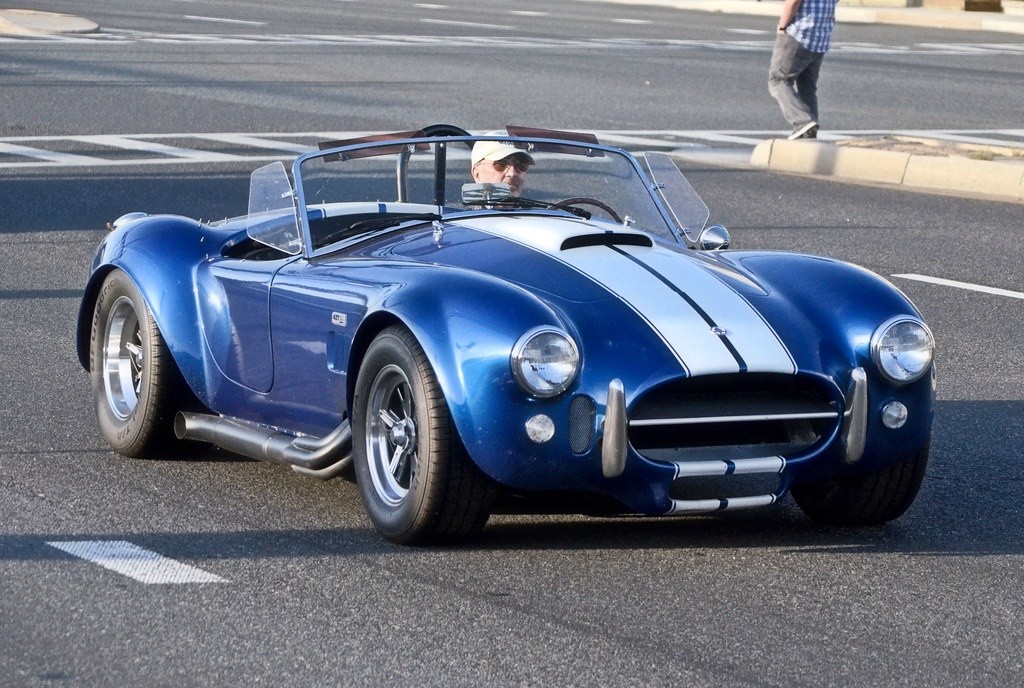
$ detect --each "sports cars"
[76,125,935,546]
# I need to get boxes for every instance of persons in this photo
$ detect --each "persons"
[471,129,536,210]
[768,0,838,141]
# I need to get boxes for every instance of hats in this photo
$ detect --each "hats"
[471,130,536,168]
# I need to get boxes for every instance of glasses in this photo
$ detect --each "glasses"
[474,157,529,173]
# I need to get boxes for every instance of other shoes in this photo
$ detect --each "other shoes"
[787,120,817,139]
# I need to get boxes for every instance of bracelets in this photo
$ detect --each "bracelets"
[776,25,785,31]
